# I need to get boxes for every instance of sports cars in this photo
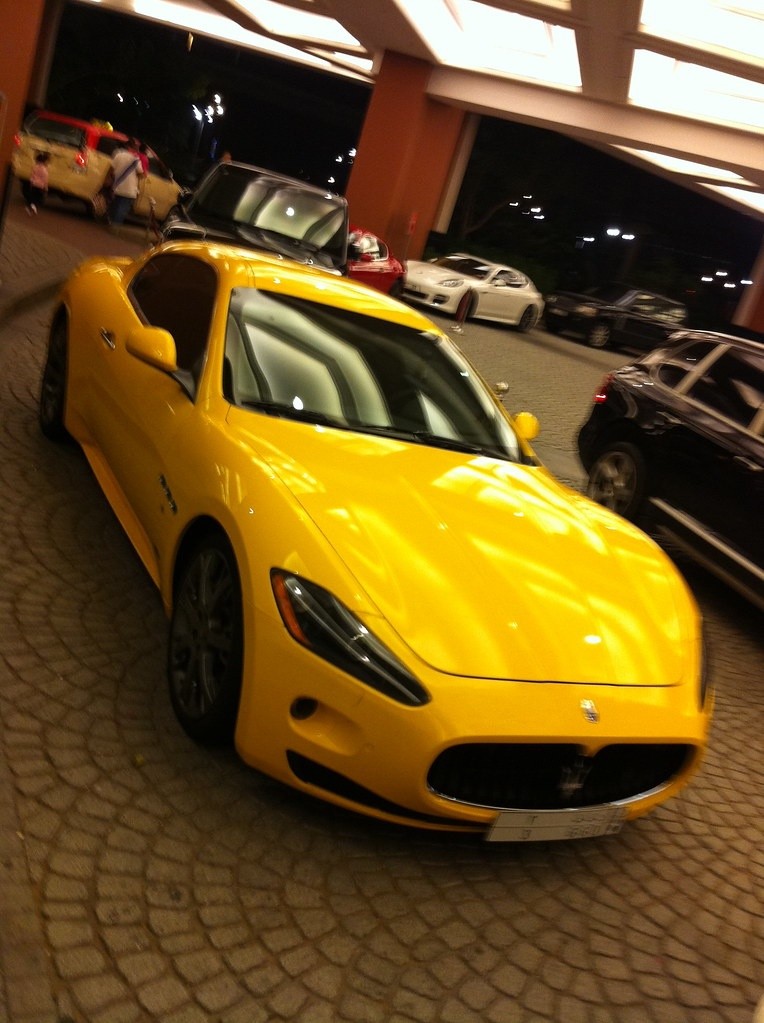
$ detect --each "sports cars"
[39,240,716,844]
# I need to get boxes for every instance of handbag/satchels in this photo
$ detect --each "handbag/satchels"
[93,192,108,216]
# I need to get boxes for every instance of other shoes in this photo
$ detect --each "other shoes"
[26,204,38,216]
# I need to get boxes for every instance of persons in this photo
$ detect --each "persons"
[24,149,51,216]
[98,138,149,225]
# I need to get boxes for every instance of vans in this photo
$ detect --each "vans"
[12,111,188,226]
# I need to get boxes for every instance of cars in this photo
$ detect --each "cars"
[401,251,545,332]
[347,230,405,299]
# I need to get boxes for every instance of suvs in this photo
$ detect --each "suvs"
[545,280,689,350]
[577,326,764,610]
[160,156,348,277]
